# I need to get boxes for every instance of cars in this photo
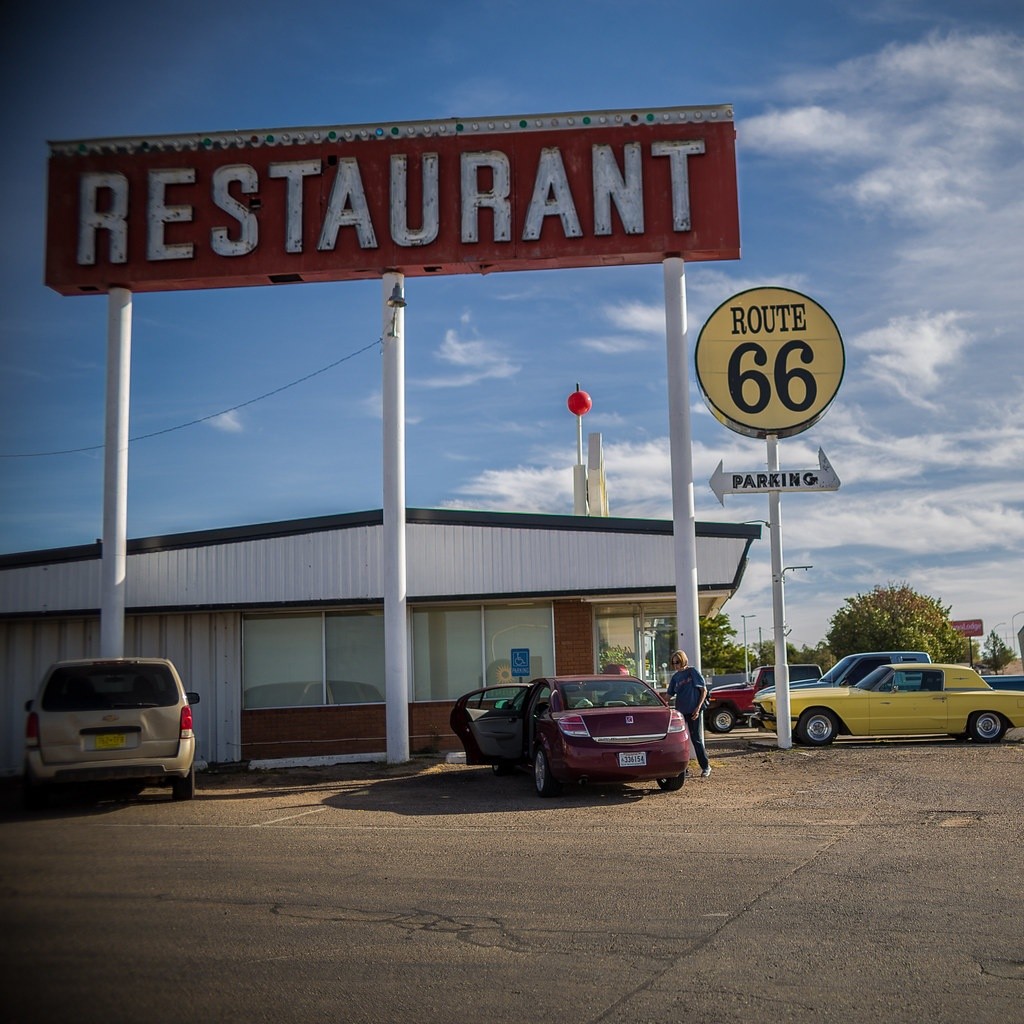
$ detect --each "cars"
[751,664,1023,746]
[24,657,202,806]
[449,672,692,797]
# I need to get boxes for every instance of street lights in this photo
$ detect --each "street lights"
[741,614,757,682]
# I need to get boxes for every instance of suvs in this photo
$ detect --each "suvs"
[704,663,824,734]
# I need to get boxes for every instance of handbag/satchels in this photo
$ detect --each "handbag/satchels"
[699,690,710,710]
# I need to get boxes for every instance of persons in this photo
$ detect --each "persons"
[664,650,711,778]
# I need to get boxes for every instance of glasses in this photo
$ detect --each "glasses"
[673,661,682,665]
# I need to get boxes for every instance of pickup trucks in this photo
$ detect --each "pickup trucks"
[754,651,1024,716]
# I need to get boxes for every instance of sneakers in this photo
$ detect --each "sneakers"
[701,764,712,776]
[686,767,689,775]
